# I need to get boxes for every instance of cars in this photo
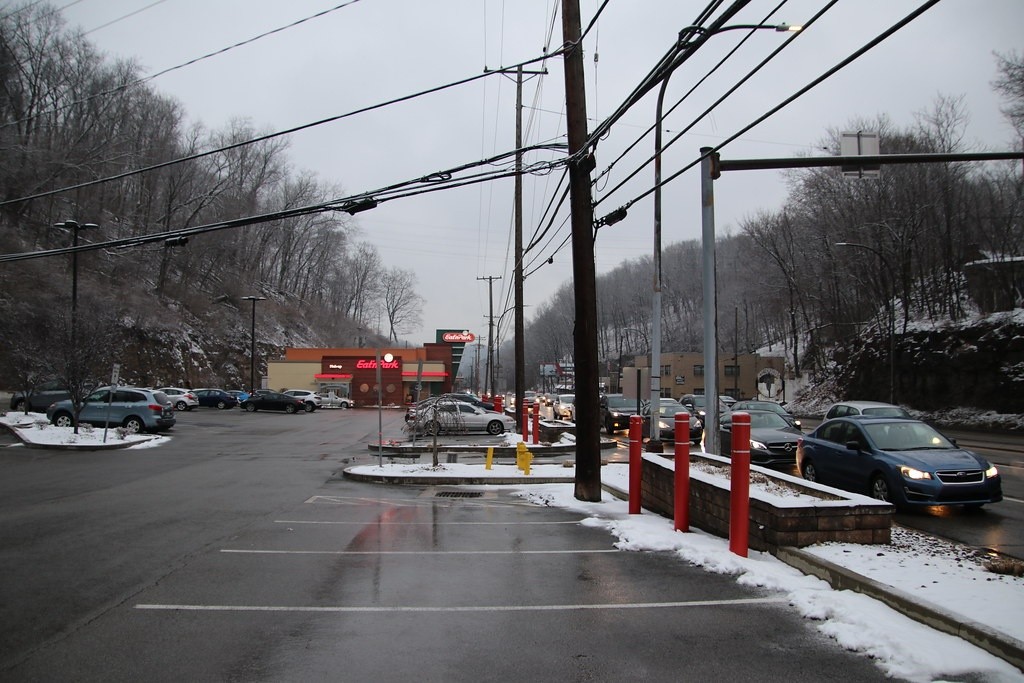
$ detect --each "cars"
[678,393,730,431]
[718,394,736,407]
[46,384,176,435]
[600,393,646,436]
[522,389,577,423]
[153,385,357,413]
[794,415,1005,516]
[697,408,809,468]
[730,401,803,433]
[817,400,945,446]
[640,400,703,447]
[9,382,99,414]
[402,390,517,436]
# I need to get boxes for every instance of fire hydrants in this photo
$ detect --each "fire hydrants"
[515,442,529,470]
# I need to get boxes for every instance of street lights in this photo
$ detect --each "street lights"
[649,21,794,459]
[623,326,652,399]
[835,241,895,403]
[496,305,532,393]
[240,295,267,395]
[52,218,100,394]
